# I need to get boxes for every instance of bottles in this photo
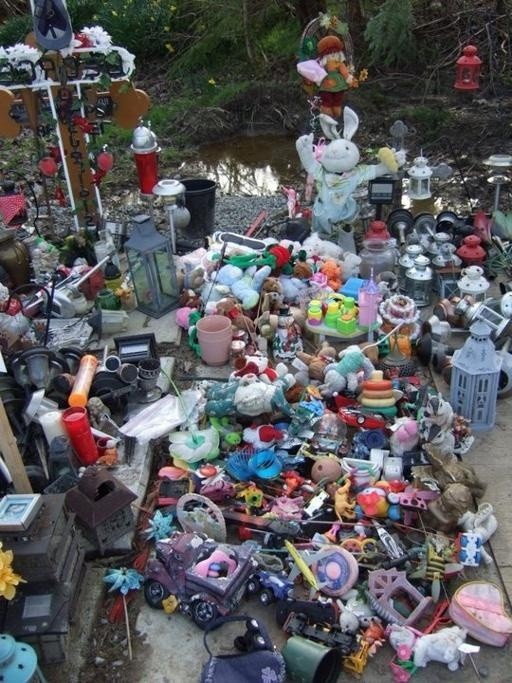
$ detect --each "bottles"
[359,237,394,281]
[229,340,247,366]
[94,289,119,311]
[69,354,99,408]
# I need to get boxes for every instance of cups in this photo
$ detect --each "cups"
[191,316,233,366]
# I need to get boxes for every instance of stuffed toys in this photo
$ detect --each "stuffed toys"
[159,233,512,671]
[296,38,406,238]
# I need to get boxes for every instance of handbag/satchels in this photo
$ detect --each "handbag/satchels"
[204,616,287,683]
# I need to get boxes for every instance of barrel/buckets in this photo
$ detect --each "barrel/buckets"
[177,177,217,239]
[195,314,232,366]
[281,633,340,683]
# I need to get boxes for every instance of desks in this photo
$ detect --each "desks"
[307,312,384,358]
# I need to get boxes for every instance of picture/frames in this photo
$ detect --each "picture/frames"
[0,493,43,533]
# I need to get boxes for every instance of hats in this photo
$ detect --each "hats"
[34,0,72,50]
